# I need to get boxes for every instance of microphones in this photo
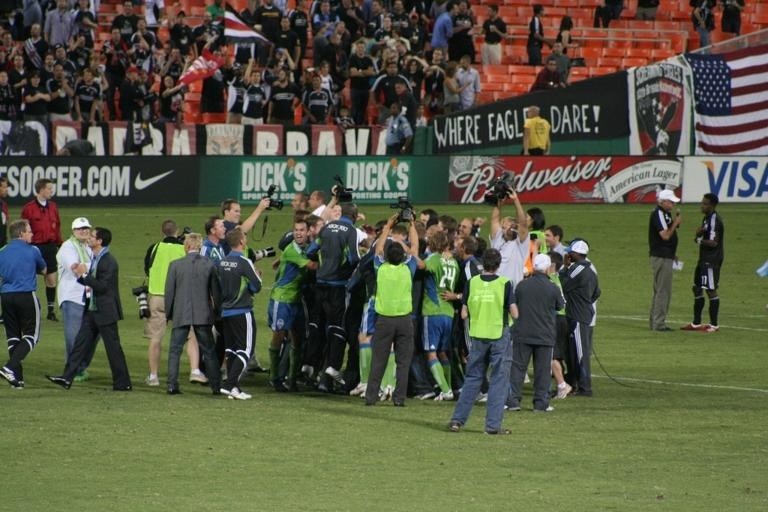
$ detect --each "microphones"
[675,208,681,229]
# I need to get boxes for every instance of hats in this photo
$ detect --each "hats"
[660,189,680,202]
[71,217,92,230]
[564,237,590,255]
[533,253,552,271]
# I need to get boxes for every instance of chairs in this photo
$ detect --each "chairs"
[92,0,767,122]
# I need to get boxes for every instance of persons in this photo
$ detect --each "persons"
[0,175,10,325]
[54,216,94,383]
[45,228,132,391]
[649,188,682,332]
[447,249,519,436]
[0,218,48,389]
[679,192,724,332]
[143,196,271,399]
[21,178,63,322]
[268,184,601,411]
[1,1,746,156]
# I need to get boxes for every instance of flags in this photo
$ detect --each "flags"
[686,44,767,156]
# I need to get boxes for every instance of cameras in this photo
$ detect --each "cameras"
[260,183,285,212]
[246,246,276,266]
[129,285,153,321]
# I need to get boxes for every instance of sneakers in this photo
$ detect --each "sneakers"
[484,427,511,436]
[47,311,59,321]
[167,388,184,394]
[393,375,592,413]
[0,365,24,389]
[653,322,719,333]
[143,375,159,386]
[247,368,269,374]
[189,371,252,400]
[266,364,367,396]
[447,421,460,432]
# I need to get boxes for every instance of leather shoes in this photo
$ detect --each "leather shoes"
[45,375,71,389]
[113,383,131,391]
